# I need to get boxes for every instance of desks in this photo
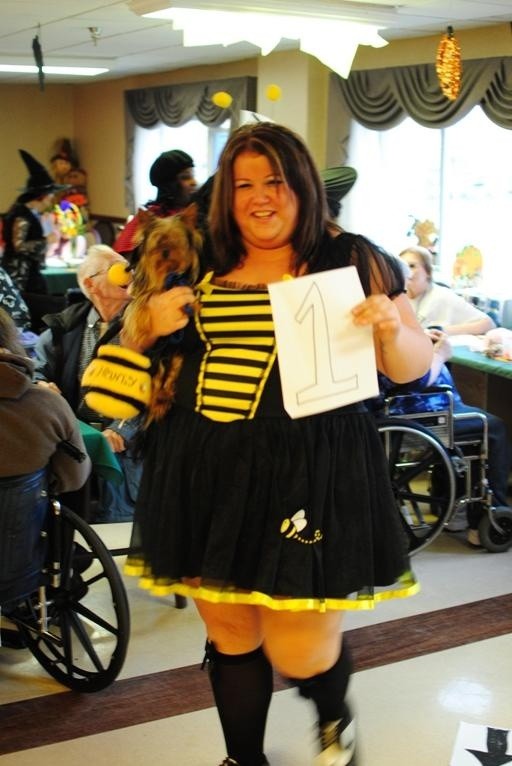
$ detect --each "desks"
[70,412,122,526]
[439,339,512,493]
[36,260,82,300]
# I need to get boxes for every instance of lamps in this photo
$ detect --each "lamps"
[125,0,401,81]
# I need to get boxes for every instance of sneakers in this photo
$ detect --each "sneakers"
[313,708,361,766]
[445,509,487,549]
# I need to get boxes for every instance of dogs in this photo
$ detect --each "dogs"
[119,202,204,432]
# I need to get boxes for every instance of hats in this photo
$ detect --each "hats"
[148,147,197,188]
[15,148,72,199]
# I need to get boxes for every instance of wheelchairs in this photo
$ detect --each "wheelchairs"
[372,383,511,560]
[2,437,132,694]
